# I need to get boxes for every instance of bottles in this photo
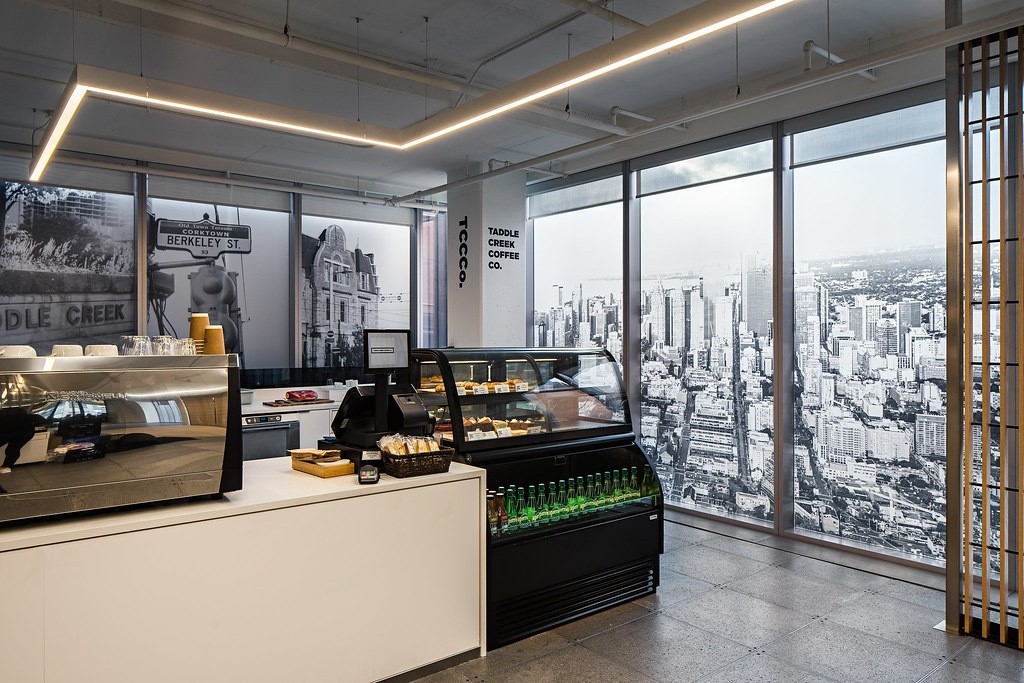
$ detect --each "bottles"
[486,464,652,537]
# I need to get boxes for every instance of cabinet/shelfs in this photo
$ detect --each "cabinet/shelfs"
[409,346,664,652]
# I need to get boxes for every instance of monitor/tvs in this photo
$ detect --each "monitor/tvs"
[331,384,411,440]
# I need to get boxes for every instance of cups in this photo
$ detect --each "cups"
[241,389,253,404]
[0,345,36,358]
[50,345,83,356]
[85,345,118,356]
[120,313,225,355]
[335,382,343,390]
[346,379,358,387]
[326,378,333,385]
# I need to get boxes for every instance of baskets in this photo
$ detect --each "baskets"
[381,445,454,478]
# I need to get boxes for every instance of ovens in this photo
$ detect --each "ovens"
[242,412,300,460]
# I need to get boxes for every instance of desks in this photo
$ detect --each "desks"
[0,455,486,683]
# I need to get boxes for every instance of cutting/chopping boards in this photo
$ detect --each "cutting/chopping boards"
[263,398,334,407]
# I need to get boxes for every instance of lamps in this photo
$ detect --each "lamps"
[29,0,793,183]
